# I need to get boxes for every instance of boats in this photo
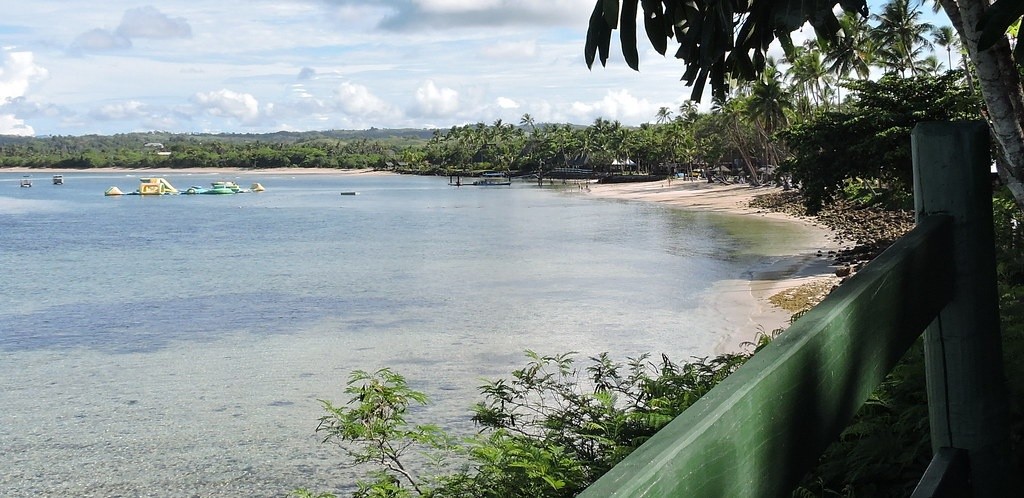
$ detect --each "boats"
[127,178,244,194]
[21,178,32,188]
[483,172,504,177]
[53,175,63,184]
